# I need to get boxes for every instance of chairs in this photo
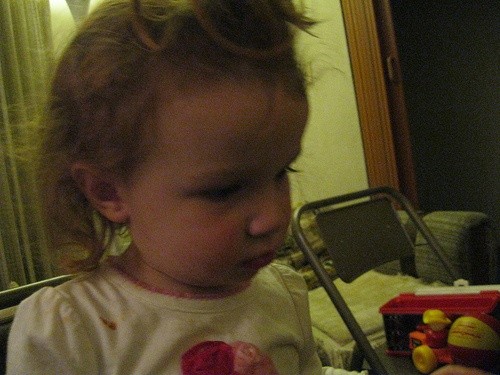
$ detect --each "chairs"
[289,184,500,375]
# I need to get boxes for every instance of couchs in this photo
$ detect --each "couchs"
[280,195,491,375]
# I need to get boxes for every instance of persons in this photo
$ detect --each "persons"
[5,0,380,375]
